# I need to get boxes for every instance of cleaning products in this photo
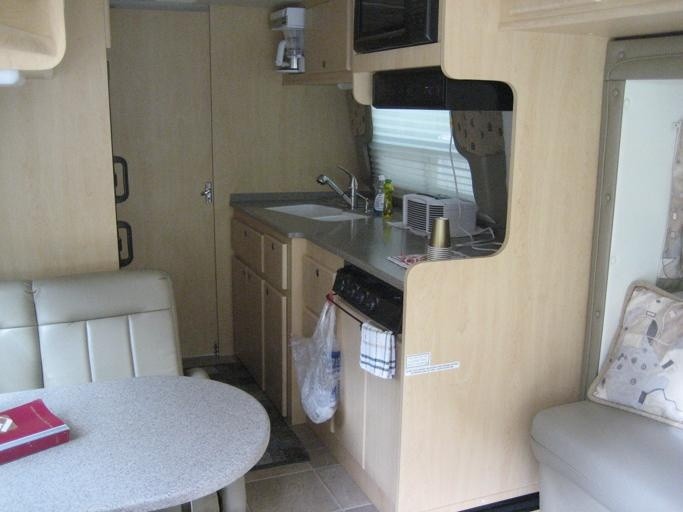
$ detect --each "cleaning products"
[371,174,393,217]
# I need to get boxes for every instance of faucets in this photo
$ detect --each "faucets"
[317,164,359,210]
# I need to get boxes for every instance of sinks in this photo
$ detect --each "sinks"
[265,202,369,222]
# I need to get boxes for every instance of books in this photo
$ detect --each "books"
[0,396,71,466]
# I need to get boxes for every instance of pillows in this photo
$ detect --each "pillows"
[587,279,681,431]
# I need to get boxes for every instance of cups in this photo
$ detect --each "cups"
[425,217,453,262]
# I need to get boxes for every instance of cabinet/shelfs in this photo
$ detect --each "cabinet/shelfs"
[297,0,354,83]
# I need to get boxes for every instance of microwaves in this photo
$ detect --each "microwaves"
[353,0,440,55]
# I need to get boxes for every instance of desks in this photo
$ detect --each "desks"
[0,374,272,510]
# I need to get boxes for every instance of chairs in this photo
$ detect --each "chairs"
[530,288,683,512]
[0,267,246,511]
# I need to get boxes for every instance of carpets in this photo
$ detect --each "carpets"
[183,362,310,472]
